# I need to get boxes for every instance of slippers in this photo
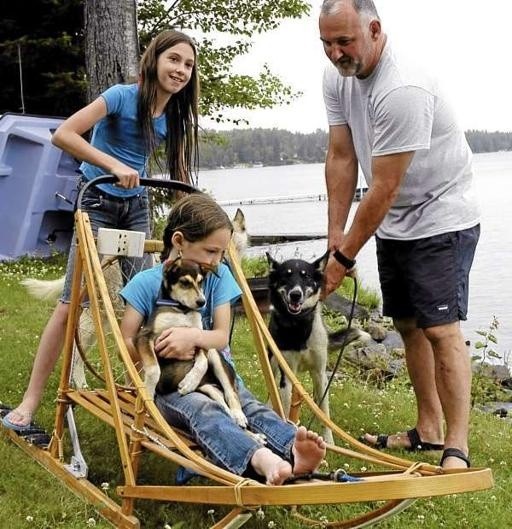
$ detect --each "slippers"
[360,427,444,450]
[1,409,35,431]
[439,447,471,468]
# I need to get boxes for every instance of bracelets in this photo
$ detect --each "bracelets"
[333,249,357,274]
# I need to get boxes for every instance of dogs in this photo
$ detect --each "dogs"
[265,248,363,448]
[16,208,249,389]
[134,257,252,430]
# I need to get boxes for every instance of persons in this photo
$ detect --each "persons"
[320,0,481,470]
[2,27,199,431]
[116,194,326,487]
